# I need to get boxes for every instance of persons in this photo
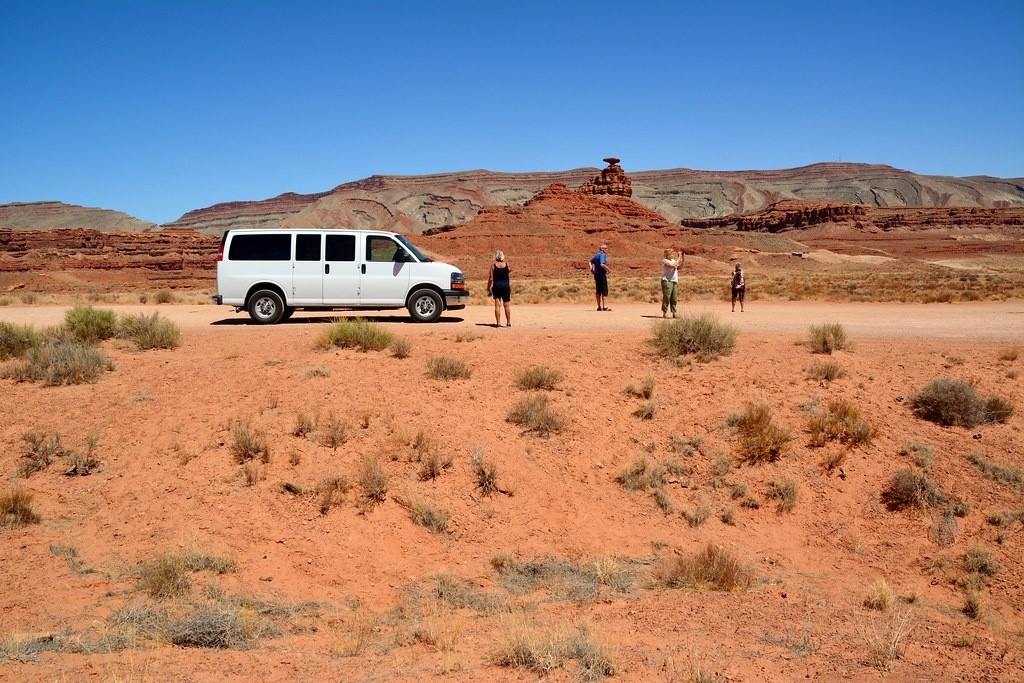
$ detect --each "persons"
[731,262,745,312]
[487,251,511,327]
[587,242,612,311]
[661,249,685,318]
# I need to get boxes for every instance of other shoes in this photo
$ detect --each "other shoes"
[507,324,511,327]
[663,314,672,319]
[597,308,602,311]
[673,313,681,318]
[603,307,611,311]
[497,323,501,327]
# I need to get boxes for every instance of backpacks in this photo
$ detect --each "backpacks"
[733,272,744,289]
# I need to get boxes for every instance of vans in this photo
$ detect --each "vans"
[212,227,470,324]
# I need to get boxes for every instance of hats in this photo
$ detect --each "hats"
[736,262,741,267]
